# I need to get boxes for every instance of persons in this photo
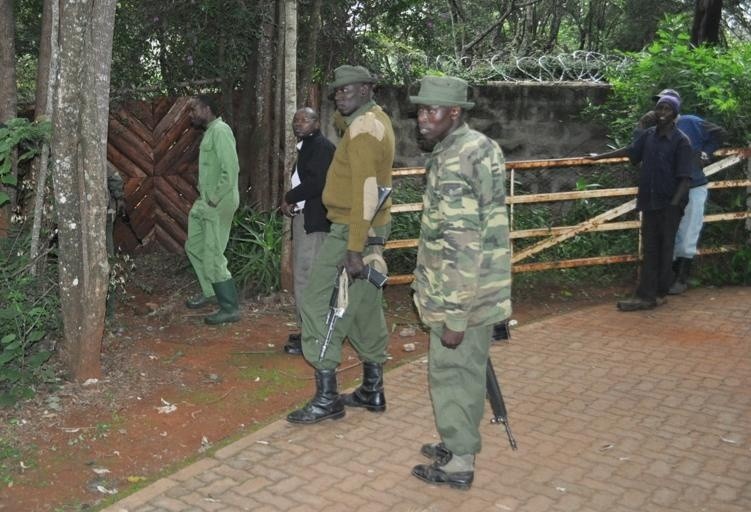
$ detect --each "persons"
[286,65,394,424]
[639,88,729,294]
[285,106,341,354]
[584,96,693,311]
[407,76,511,494]
[185,93,240,323]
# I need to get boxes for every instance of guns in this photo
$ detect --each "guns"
[486,320,517,450]
[319,185,392,361]
[119,210,143,245]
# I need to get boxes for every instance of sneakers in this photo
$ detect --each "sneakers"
[617,295,668,310]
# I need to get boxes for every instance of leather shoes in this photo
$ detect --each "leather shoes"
[284,332,303,355]
[412,442,473,491]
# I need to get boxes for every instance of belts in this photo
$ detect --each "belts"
[293,209,303,215]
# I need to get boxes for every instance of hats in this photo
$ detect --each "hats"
[328,65,377,91]
[409,76,475,112]
[652,88,682,115]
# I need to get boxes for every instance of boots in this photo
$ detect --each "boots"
[187,278,240,325]
[667,257,688,294]
[287,369,345,425]
[341,361,387,411]
